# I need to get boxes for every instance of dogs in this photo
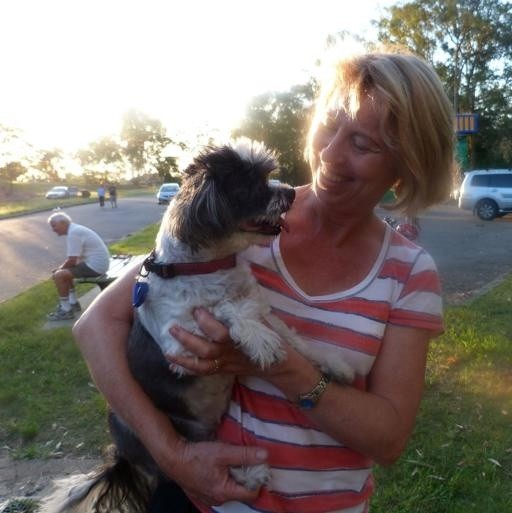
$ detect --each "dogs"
[32,136,297,513]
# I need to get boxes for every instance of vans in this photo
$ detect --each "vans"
[458,167,512,220]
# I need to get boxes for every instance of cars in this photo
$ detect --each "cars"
[45,185,69,199]
[157,180,180,203]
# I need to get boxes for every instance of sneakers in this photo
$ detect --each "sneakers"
[71,300,83,313]
[45,303,75,321]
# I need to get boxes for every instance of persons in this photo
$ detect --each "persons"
[108,182,117,207]
[69,40,458,512]
[97,184,105,206]
[48,205,110,320]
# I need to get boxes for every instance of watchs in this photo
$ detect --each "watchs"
[287,374,330,412]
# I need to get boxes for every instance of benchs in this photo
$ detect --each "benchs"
[76,249,152,297]
